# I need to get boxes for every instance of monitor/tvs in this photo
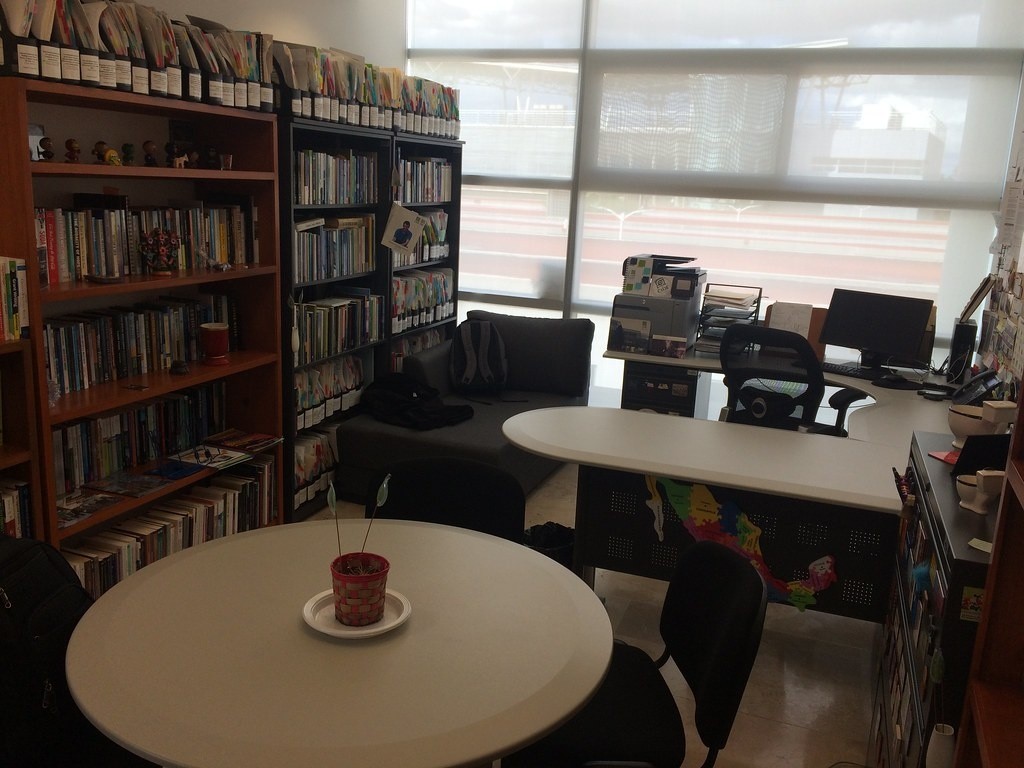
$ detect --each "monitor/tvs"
[818,289,933,371]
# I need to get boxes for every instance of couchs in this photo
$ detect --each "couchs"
[336,312,593,503]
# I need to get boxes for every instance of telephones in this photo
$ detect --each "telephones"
[951,368,1003,406]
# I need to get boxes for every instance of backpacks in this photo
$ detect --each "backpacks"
[447,318,508,398]
[0,534,96,732]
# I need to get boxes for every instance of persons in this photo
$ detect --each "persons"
[392,220,412,246]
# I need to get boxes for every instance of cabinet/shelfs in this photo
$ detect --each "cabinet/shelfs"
[865,430,1001,768]
[952,358,1024,768]
[0,73,464,626]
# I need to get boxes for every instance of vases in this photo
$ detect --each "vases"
[926,649,955,768]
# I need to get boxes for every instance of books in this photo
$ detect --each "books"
[295,214,376,283]
[396,147,453,202]
[1,0,276,82]
[295,346,367,430]
[206,427,284,453]
[960,274,997,323]
[51,379,229,497]
[294,145,381,205]
[41,291,244,394]
[396,327,442,354]
[392,208,450,265]
[272,39,460,121]
[33,192,261,284]
[61,455,276,601]
[0,255,31,341]
[0,479,36,540]
[293,288,384,367]
[168,444,254,470]
[391,267,452,317]
[294,422,344,492]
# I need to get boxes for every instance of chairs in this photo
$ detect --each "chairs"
[719,323,867,438]
[499,540,766,768]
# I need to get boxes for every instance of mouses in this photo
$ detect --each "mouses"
[881,375,904,382]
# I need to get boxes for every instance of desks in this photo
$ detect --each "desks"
[64,518,614,768]
[502,350,965,623]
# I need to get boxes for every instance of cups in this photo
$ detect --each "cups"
[200,323,229,365]
[221,155,232,171]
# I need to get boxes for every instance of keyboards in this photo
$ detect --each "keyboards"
[820,358,889,381]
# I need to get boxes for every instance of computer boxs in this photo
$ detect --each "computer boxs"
[946,318,977,384]
